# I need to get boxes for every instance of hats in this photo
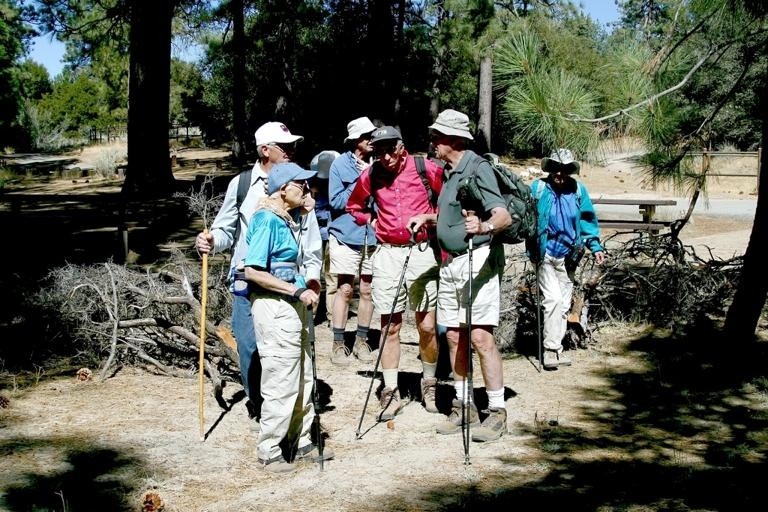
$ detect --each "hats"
[541,148,580,175]
[344,117,377,144]
[428,109,474,140]
[369,126,402,145]
[268,163,319,196]
[255,122,304,146]
[310,151,341,179]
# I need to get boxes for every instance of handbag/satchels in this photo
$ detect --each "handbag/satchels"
[268,262,298,282]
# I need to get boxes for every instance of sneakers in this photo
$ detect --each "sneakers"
[376,385,402,422]
[436,399,481,435]
[558,353,571,366]
[293,443,335,463]
[472,406,508,442]
[543,349,559,369]
[257,454,297,476]
[330,341,350,367]
[420,378,445,413]
[353,335,373,364]
[249,413,261,433]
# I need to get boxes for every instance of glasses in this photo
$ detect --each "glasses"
[546,164,571,174]
[373,144,401,159]
[266,144,294,152]
[287,182,307,196]
[429,134,449,143]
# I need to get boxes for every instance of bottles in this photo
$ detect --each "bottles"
[566,237,587,272]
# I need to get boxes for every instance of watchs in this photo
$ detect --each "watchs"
[484,221,495,236]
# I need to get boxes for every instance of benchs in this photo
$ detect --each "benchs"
[598,220,664,241]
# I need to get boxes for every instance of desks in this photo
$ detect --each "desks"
[592,195,678,224]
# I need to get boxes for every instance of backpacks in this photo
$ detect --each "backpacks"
[470,153,536,244]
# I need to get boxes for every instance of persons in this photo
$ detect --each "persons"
[526,148,607,369]
[309,151,342,329]
[328,115,376,365]
[196,121,324,422]
[243,163,333,474]
[346,125,446,422]
[422,140,452,381]
[406,108,513,442]
